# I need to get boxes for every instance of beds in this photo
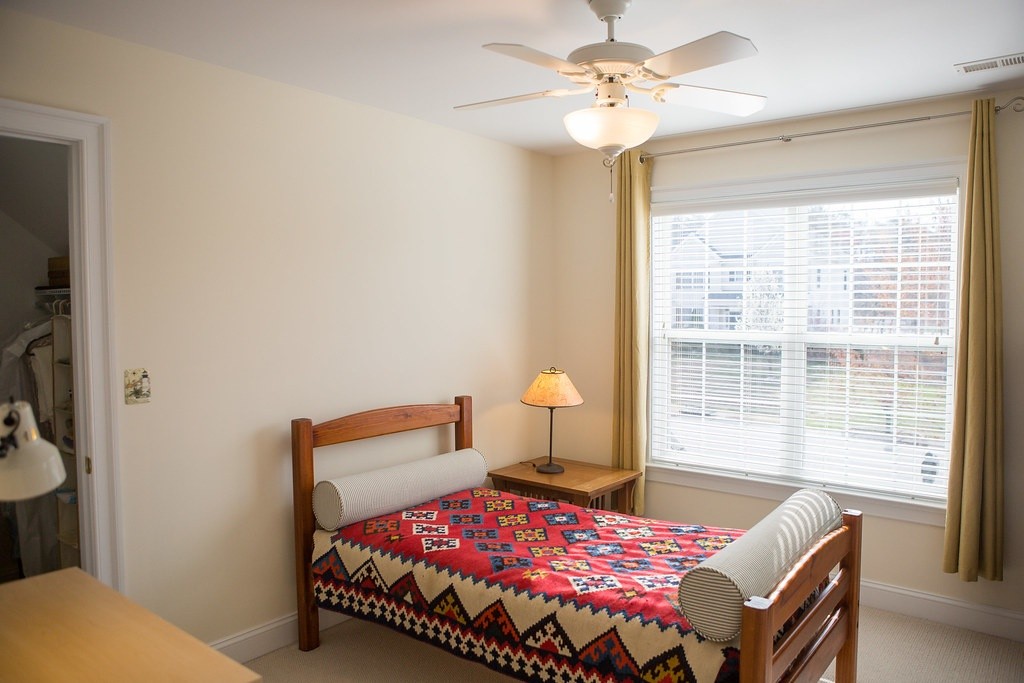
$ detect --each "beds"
[291,395,864,683]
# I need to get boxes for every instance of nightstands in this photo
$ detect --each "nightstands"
[486,455,643,515]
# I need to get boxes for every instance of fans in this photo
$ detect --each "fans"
[452,0,768,117]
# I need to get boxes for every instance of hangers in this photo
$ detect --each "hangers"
[23,299,70,356]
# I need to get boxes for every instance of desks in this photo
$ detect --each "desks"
[0,566,263,683]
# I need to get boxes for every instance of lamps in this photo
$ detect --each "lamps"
[0,394,68,502]
[564,83,660,203]
[519,367,584,474]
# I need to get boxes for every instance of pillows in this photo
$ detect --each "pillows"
[678,488,843,644]
[311,446,489,532]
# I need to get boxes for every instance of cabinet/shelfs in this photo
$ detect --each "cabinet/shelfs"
[52,313,82,569]
